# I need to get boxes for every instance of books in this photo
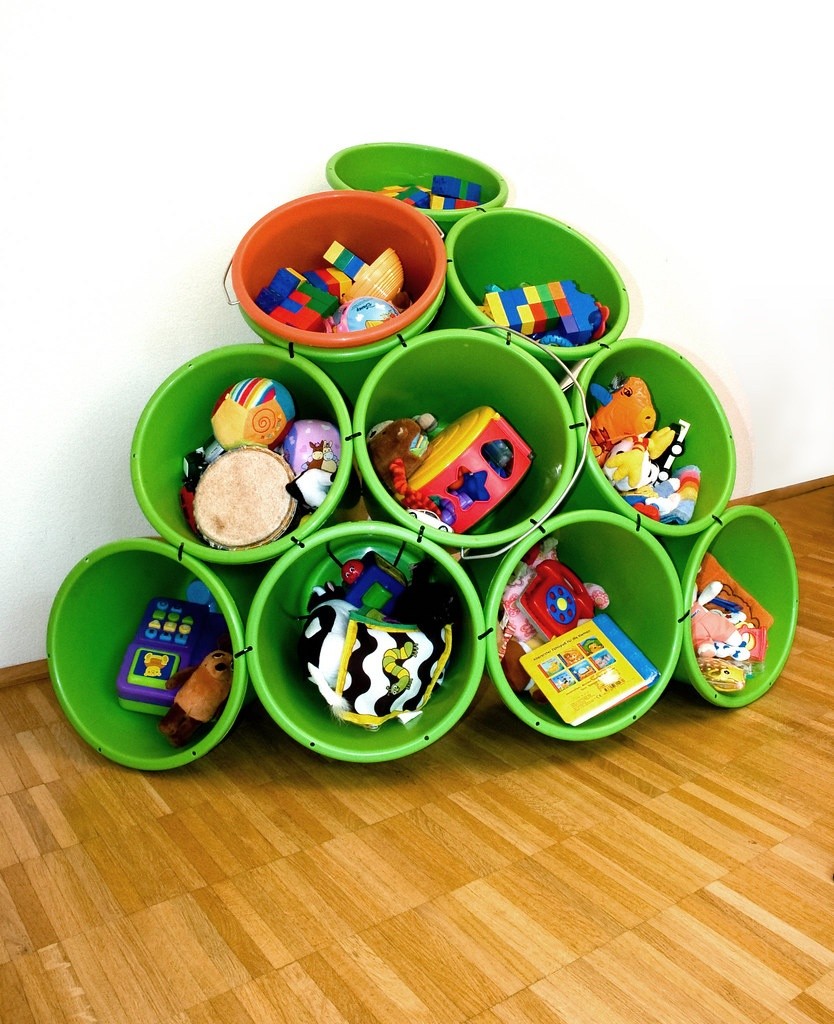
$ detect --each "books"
[519,614,661,726]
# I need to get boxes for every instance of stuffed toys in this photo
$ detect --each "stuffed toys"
[690,552,774,661]
[603,424,701,525]
[297,586,455,728]
[496,537,609,716]
[211,377,341,514]
[367,413,439,495]
[158,650,233,748]
